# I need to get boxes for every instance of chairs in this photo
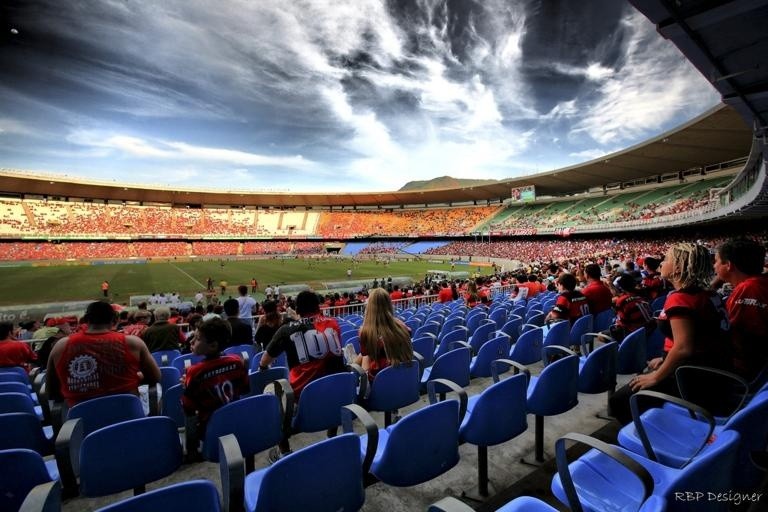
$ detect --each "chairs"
[0,292,768,512]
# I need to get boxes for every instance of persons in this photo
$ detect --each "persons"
[1,176,766,390]
[606,244,732,427]
[259,290,347,467]
[178,316,252,459]
[45,300,161,406]
